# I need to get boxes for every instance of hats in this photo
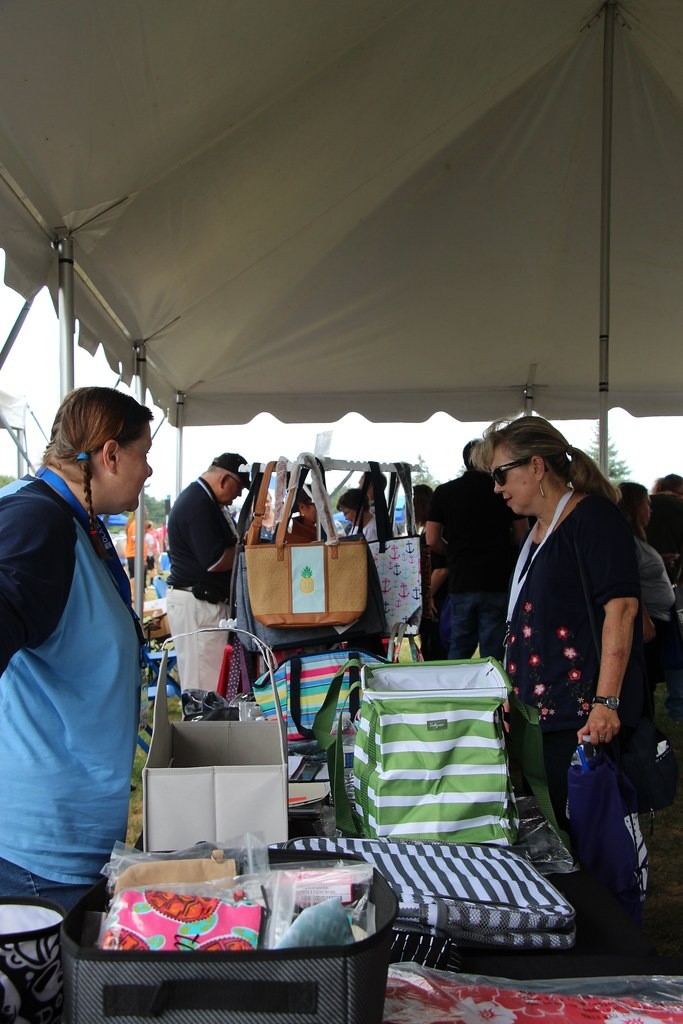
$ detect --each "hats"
[212,454,250,490]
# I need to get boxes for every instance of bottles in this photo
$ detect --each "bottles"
[565,734,592,819]
[335,520,347,537]
[329,710,356,806]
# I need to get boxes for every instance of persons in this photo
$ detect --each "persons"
[0,384,155,933]
[125,504,167,604]
[470,416,648,869]
[249,438,537,664]
[612,475,683,724]
[165,451,252,723]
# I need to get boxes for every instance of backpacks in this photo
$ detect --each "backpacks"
[268,837,578,950]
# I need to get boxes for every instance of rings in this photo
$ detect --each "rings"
[599,735,606,739]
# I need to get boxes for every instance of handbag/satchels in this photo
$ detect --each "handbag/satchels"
[620,716,679,815]
[252,648,392,742]
[650,613,683,670]
[312,656,575,865]
[60,846,398,1024]
[181,453,424,721]
[140,617,288,852]
[567,744,648,929]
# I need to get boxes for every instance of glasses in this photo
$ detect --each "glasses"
[488,457,530,487]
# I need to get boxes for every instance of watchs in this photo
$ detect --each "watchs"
[594,696,620,710]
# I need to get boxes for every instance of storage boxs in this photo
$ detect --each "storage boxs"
[352,657,520,845]
[59,849,399,1024]
[142,599,169,638]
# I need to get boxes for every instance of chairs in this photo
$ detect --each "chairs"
[153,576,167,598]
[159,552,171,572]
[143,646,181,697]
